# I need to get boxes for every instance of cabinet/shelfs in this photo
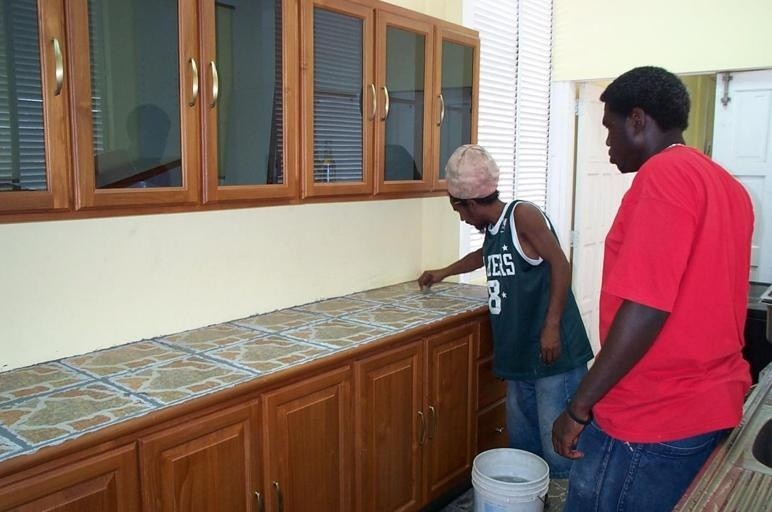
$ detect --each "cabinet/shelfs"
[1,0,73,214]
[299,0,433,204]
[353,322,473,512]
[70,0,303,219]
[138,367,353,512]
[435,18,481,195]
[1,440,138,512]
[476,314,507,452]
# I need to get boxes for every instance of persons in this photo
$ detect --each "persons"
[552,66,754,512]
[94,104,174,189]
[418,144,594,480]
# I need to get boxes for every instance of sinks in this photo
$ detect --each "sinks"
[729,366,772,475]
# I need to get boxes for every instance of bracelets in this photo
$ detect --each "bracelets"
[564,399,593,425]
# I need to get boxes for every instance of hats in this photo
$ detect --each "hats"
[446,144,501,200]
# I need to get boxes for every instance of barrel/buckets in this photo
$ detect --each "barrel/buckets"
[470,448,550,511]
